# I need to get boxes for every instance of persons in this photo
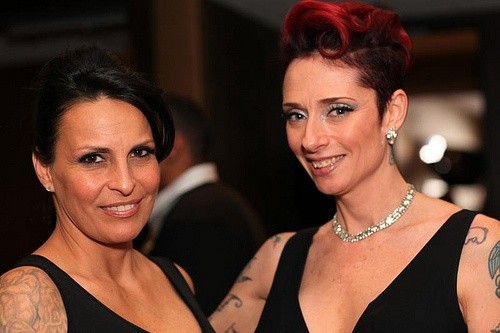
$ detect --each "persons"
[149,92,266,318]
[0,42,217,333]
[173,0,500,333]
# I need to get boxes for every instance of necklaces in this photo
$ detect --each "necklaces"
[331,183,416,243]
